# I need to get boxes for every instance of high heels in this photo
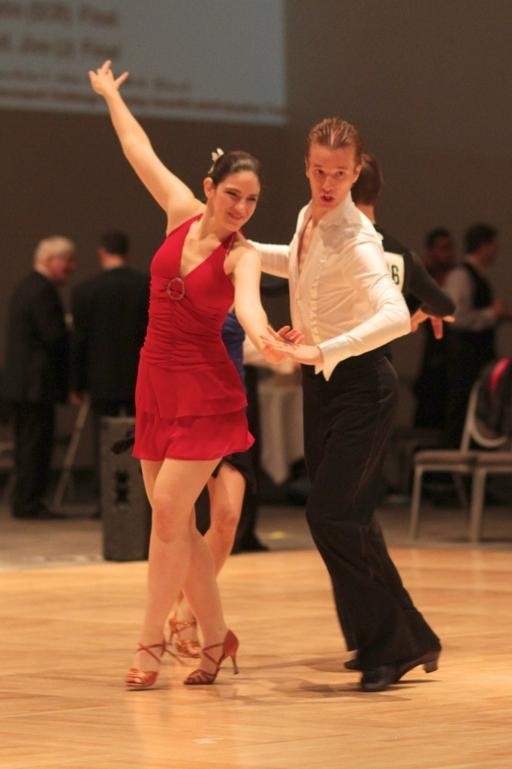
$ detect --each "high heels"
[124,630,165,688]
[183,628,240,685]
[169,611,202,658]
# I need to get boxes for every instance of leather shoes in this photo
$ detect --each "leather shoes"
[343,630,442,691]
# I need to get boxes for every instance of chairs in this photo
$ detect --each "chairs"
[407,359,511,548]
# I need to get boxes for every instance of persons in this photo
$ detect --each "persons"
[9,227,152,519]
[405,223,504,498]
[86,59,306,692]
[246,116,456,692]
